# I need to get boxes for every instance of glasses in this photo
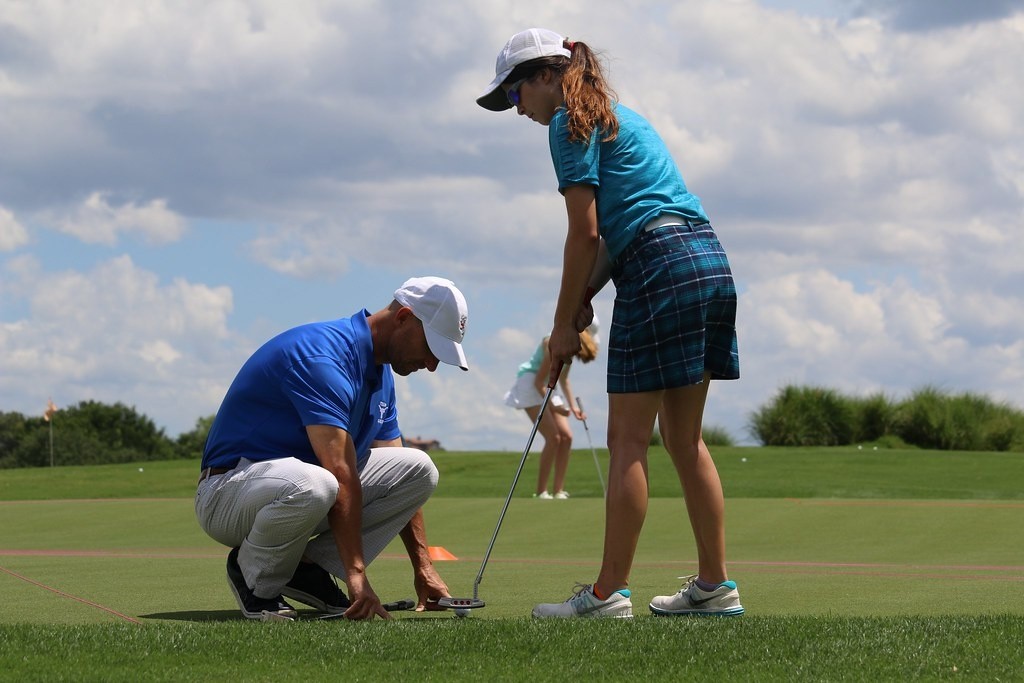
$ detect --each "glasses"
[506,77,528,106]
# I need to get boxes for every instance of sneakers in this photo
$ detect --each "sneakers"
[531,581,633,618]
[225,547,295,619]
[533,491,553,499]
[281,561,352,614]
[555,491,570,500]
[649,574,745,616]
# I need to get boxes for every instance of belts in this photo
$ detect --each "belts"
[640,217,706,236]
[197,466,235,486]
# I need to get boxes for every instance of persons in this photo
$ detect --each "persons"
[505,312,600,498]
[477,26,745,620]
[195,276,470,621]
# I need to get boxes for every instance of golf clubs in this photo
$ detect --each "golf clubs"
[261,599,415,625]
[437,361,564,609]
[575,397,607,497]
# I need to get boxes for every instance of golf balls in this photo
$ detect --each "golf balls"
[453,609,471,617]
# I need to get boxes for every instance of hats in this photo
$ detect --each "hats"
[393,276,469,371]
[585,312,601,344]
[475,27,572,112]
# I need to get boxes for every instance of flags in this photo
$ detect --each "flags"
[44,398,56,421]
